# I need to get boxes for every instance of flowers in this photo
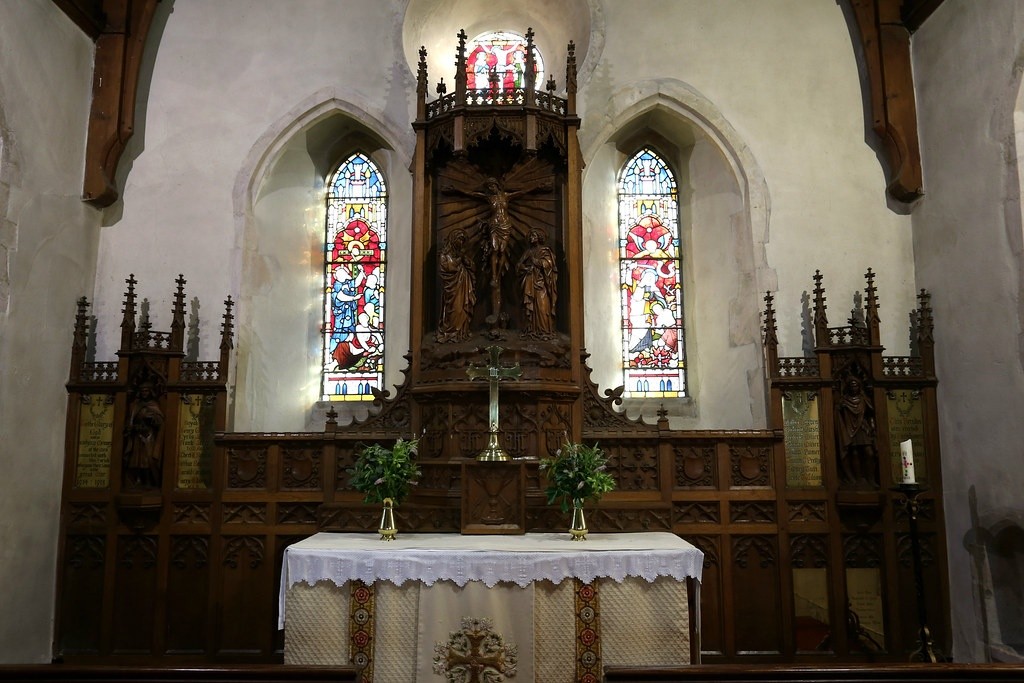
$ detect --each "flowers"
[347,429,426,507]
[538,431,616,514]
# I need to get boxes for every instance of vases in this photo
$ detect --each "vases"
[569,497,588,541]
[379,498,398,541]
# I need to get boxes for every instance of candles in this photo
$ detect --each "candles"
[900,439,915,483]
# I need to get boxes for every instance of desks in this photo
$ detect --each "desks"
[278,532,704,683]
